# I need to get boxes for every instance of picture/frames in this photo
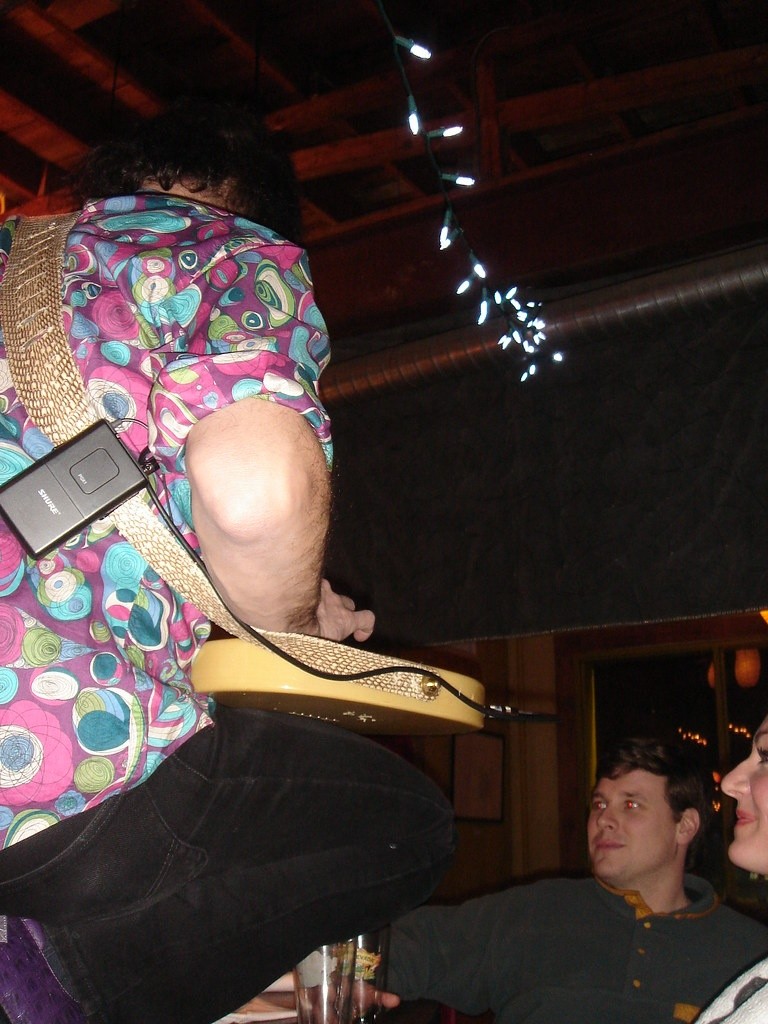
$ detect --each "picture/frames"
[446,730,508,825]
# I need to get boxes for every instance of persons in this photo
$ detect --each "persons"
[313,717,768,1024]
[0,94,455,1024]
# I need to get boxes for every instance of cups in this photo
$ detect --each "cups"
[292,937,356,1024]
[349,932,382,1024]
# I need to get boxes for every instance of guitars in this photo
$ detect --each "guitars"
[188,637,488,739]
